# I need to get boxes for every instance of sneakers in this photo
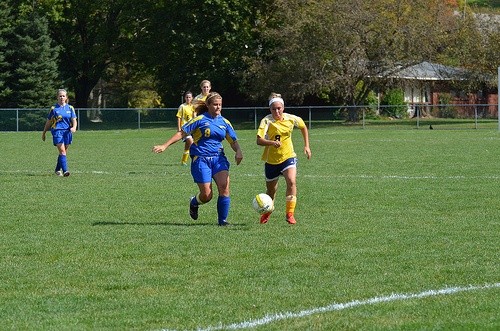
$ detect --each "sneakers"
[260,212,270,224]
[286,212,296,224]
[219,221,229,225]
[190,196,198,220]
[64,171,70,176]
[55,169,62,176]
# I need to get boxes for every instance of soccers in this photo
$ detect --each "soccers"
[252,193,273,215]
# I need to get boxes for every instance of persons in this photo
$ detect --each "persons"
[177,91,194,165]
[42,89,77,177]
[152,92,243,226]
[256,93,311,223]
[192,80,211,119]
